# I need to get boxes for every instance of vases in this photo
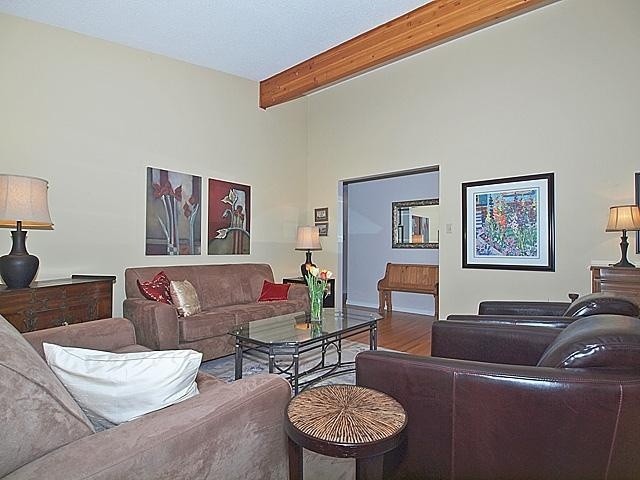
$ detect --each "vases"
[310,321,323,340]
[309,283,325,323]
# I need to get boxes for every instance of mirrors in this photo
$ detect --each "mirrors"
[390,198,438,249]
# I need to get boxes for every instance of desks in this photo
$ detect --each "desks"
[280,384,409,480]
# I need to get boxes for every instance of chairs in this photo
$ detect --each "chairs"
[0,315,294,480]
[446,290,640,322]
[351,313,640,479]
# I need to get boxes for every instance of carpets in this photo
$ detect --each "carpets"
[199,335,410,480]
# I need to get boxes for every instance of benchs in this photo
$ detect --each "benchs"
[375,260,439,322]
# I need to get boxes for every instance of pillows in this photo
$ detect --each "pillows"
[135,268,172,303]
[257,278,293,301]
[42,340,205,432]
[168,278,203,319]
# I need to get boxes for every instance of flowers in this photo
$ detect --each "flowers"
[304,263,336,317]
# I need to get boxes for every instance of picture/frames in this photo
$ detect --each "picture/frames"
[314,206,329,223]
[314,221,329,237]
[460,169,558,272]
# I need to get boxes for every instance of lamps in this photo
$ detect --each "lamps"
[606,202,639,266]
[293,225,324,279]
[0,173,57,290]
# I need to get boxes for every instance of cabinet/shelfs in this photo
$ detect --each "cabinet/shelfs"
[587,261,640,296]
[282,277,336,310]
[0,274,118,338]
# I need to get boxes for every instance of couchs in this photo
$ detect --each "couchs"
[122,262,310,364]
